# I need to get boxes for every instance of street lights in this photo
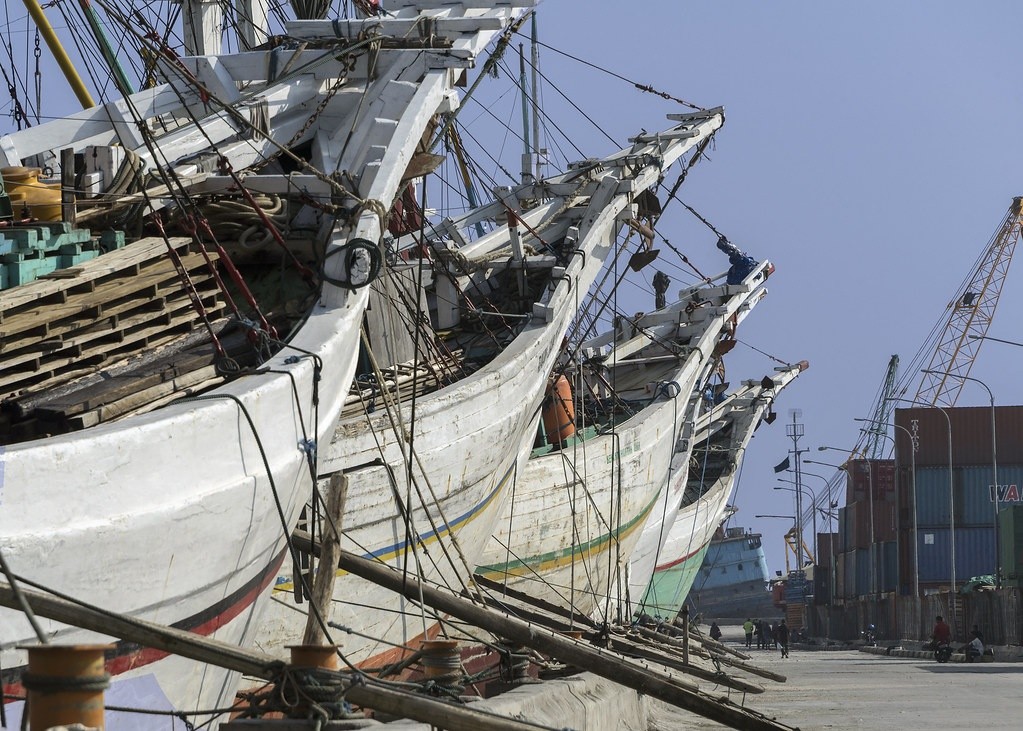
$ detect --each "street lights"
[801,458,857,595]
[881,397,957,639]
[776,478,817,564]
[816,445,876,593]
[756,515,800,575]
[861,428,902,641]
[785,469,837,607]
[919,369,1004,590]
[853,417,919,641]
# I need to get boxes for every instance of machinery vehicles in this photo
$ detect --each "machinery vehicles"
[856,353,900,460]
[782,194,1023,565]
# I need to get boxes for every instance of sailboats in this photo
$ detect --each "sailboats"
[0,1,805,731]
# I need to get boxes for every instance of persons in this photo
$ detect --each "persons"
[710,623,721,641]
[930,616,951,655]
[743,618,779,651]
[968,624,984,644]
[776,619,790,658]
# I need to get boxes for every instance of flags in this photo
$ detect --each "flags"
[774,455,790,473]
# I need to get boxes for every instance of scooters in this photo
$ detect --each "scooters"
[861,623,878,647]
[927,632,953,664]
